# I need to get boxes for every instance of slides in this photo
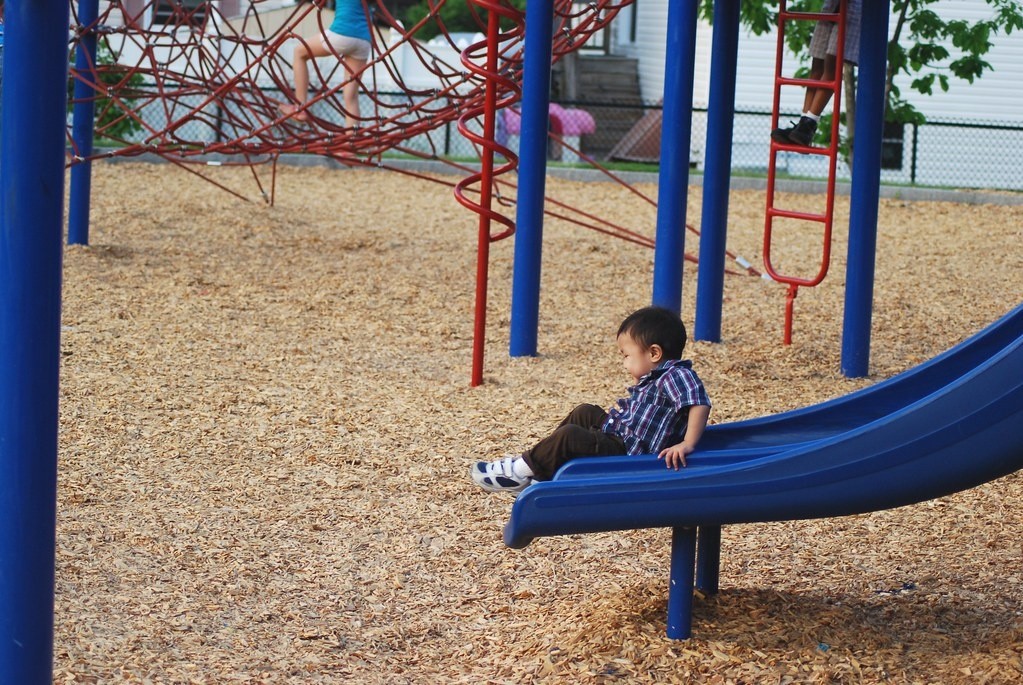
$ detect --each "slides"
[501,303,1023,551]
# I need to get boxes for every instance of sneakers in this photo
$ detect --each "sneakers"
[770,120,810,155]
[788,116,818,148]
[471,459,531,494]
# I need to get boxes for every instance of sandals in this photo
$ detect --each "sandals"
[279,103,306,123]
[334,152,356,158]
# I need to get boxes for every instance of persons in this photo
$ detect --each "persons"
[771,0,862,155]
[470,308,712,498]
[279,0,376,158]
